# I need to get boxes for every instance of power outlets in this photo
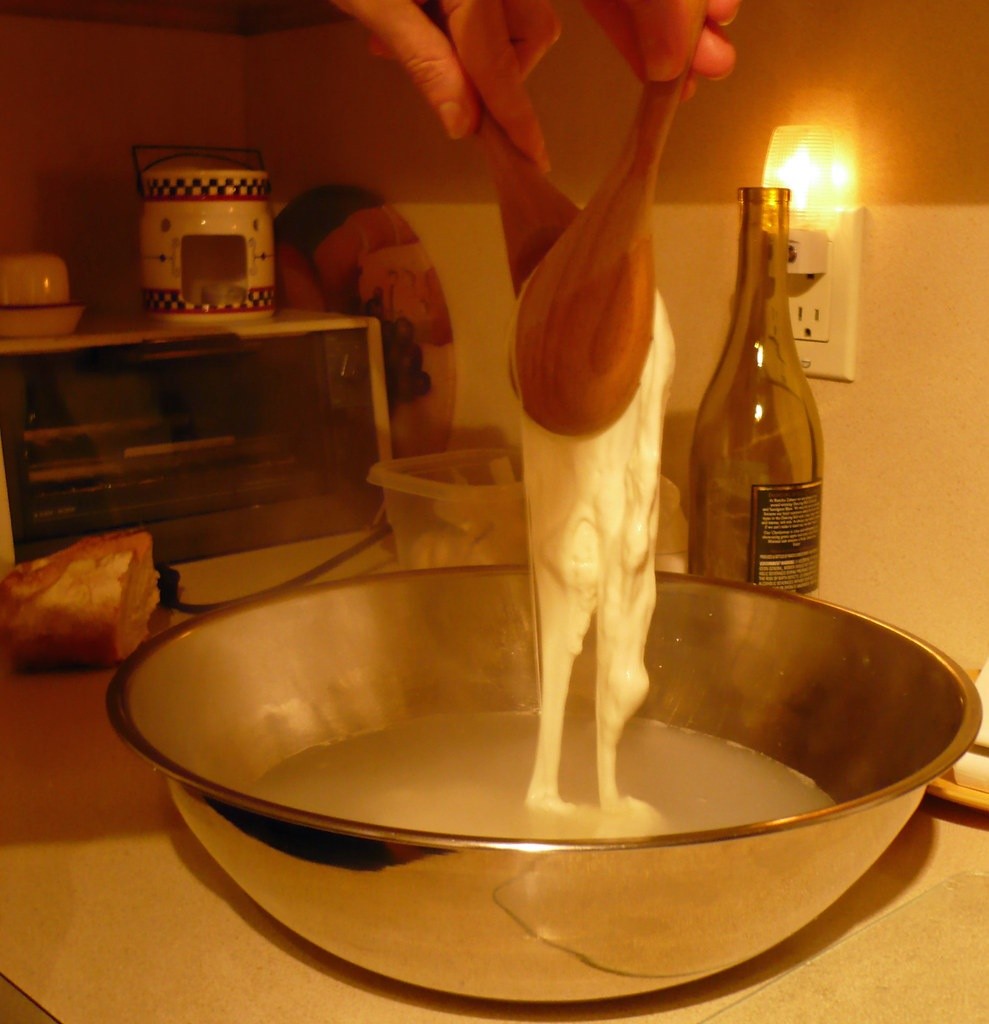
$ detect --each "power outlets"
[784,210,860,384]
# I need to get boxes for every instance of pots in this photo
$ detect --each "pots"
[107,560,983,1007]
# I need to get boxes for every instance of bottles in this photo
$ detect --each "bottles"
[689,188,823,598]
[137,170,275,320]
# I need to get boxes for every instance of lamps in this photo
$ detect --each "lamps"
[761,121,847,275]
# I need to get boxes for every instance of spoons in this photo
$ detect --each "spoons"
[476,121,581,292]
[515,17,708,441]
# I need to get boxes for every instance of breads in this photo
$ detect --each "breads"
[0,530,161,669]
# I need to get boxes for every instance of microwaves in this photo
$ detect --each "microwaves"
[1,306,394,577]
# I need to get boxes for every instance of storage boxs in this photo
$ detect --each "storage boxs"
[364,449,530,568]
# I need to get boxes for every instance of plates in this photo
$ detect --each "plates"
[925,667,988,814]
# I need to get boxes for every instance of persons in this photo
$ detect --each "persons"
[0,0,743,174]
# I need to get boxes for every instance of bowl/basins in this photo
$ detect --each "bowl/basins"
[1,304,88,337]
